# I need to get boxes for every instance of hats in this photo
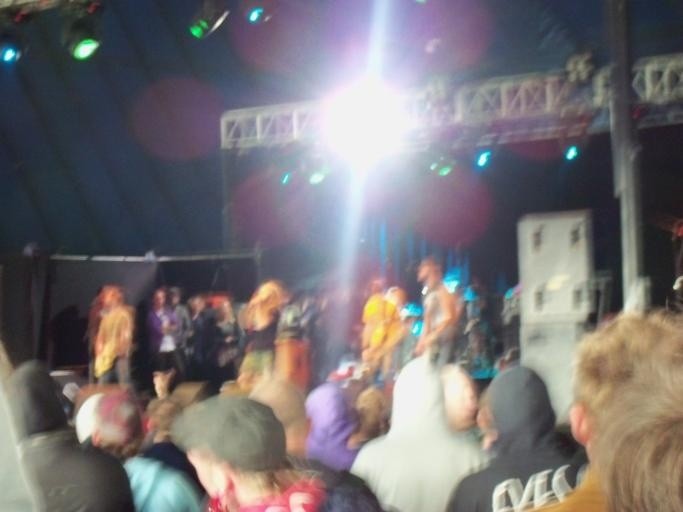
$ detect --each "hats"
[168,392,286,470]
[75,392,142,446]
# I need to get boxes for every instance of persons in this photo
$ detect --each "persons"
[1,252,682,511]
